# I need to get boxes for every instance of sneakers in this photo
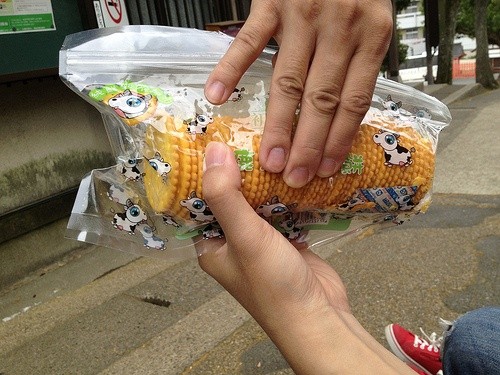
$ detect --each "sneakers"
[384,323,445,375]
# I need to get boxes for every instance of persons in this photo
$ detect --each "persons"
[191,1,500,375]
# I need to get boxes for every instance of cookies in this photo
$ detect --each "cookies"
[144,114,435,221]
